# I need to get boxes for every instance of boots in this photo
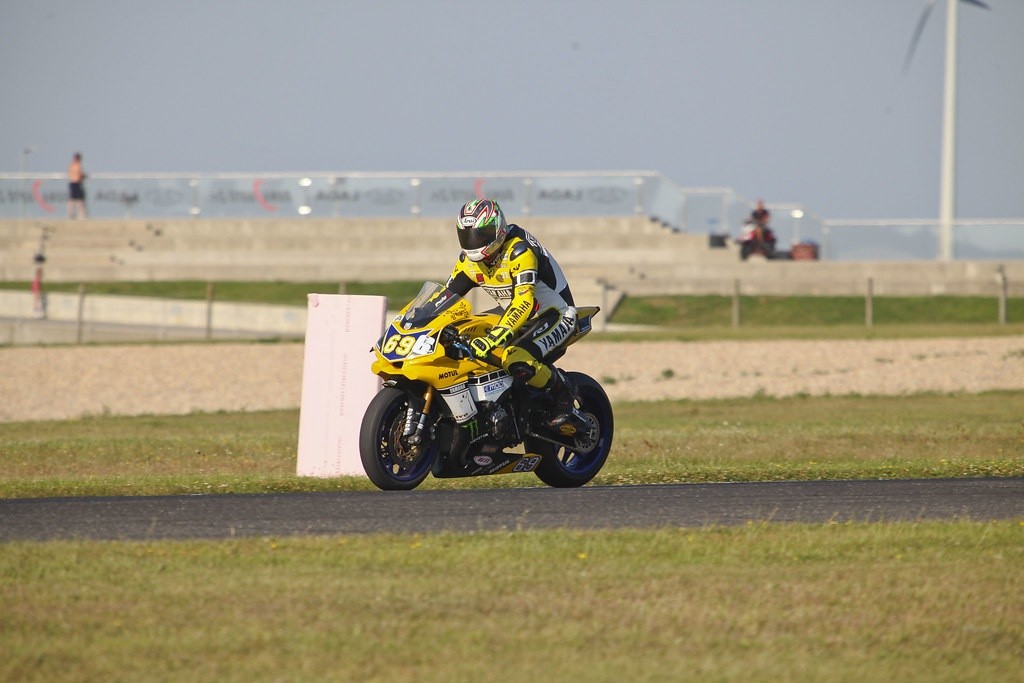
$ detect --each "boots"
[545,364,574,427]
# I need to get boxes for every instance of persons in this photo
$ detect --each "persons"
[69,153,88,218]
[411,200,579,409]
[746,200,775,249]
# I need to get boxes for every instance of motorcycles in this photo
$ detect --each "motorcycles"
[358,281,614,491]
[738,217,778,260]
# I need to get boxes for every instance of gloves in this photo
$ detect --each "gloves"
[470,328,514,360]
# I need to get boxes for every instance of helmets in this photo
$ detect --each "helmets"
[457,200,507,262]
